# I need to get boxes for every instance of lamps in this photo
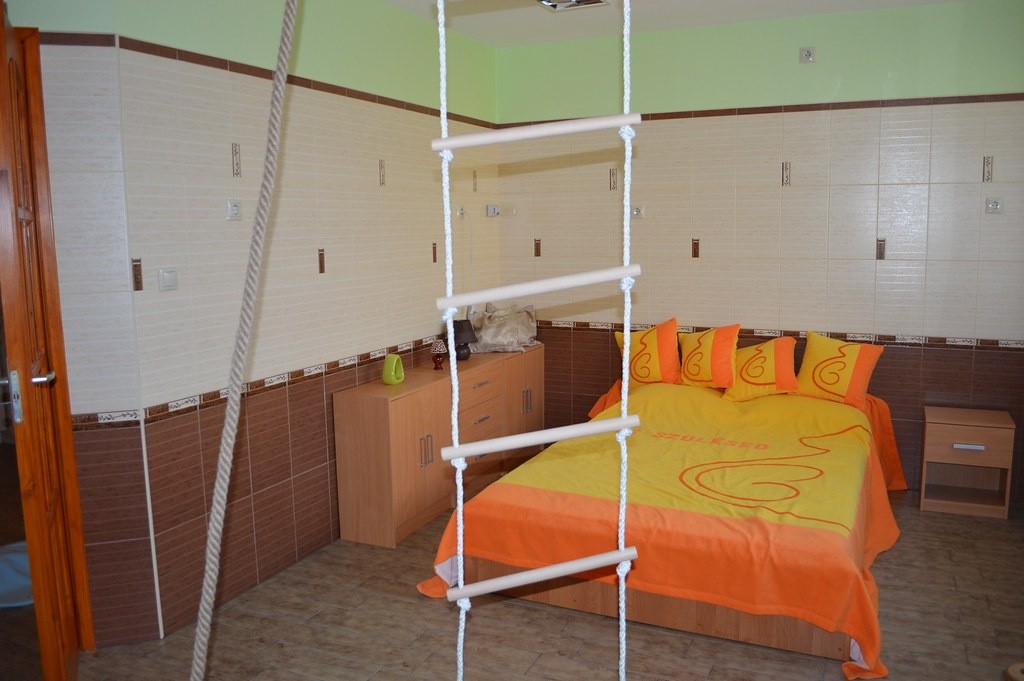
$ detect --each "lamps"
[430,340,447,370]
[453,320,478,361]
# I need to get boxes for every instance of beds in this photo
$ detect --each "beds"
[416,378,908,680]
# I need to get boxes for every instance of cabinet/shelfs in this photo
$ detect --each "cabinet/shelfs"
[333,341,544,548]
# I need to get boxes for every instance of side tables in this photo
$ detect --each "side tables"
[920,406,1016,519]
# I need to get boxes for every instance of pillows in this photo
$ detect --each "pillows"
[678,324,742,388]
[614,316,683,391]
[721,336,801,401]
[787,330,884,410]
